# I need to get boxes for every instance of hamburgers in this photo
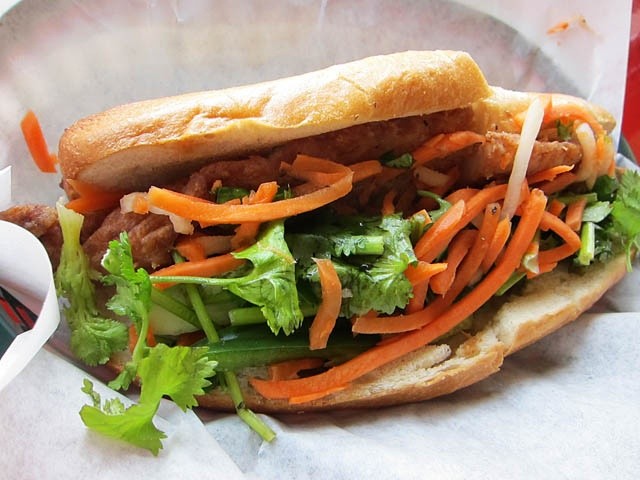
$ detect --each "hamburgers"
[57,49,639,418]
